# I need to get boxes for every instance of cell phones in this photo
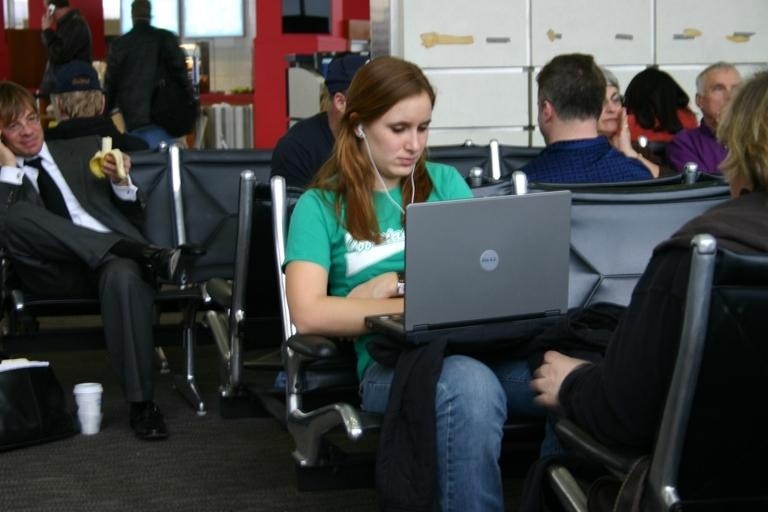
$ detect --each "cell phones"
[48,4,56,17]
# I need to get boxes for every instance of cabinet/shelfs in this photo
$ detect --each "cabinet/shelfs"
[403,0,530,148]
[654,0,767,126]
[530,0,654,147]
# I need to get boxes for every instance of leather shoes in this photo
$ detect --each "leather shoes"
[151,248,182,282]
[130,401,168,439]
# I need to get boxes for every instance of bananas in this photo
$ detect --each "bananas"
[89,136,126,179]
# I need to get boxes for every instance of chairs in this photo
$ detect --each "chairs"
[508,162,735,310]
[0,143,211,418]
[542,230,767,512]
[266,171,542,492]
[200,167,281,404]
[168,143,274,418]
[494,140,544,180]
[425,139,496,180]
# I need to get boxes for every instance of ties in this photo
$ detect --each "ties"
[24,158,72,220]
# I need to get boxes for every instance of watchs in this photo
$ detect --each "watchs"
[394,271,407,297]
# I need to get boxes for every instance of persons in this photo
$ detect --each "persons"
[505,52,743,184]
[39,1,201,153]
[278,57,614,512]
[533,69,767,509]
[268,50,372,182]
[0,82,192,440]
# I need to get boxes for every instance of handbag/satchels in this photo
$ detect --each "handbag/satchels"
[0,366,66,454]
[150,80,198,135]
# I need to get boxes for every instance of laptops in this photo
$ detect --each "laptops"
[364,189,571,346]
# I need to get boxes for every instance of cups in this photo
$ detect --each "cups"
[74,382,104,433]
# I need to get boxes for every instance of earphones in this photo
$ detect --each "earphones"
[357,123,364,135]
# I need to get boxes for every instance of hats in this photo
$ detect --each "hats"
[326,51,371,92]
[56,61,103,93]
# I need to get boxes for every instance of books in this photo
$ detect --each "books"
[191,101,254,149]
